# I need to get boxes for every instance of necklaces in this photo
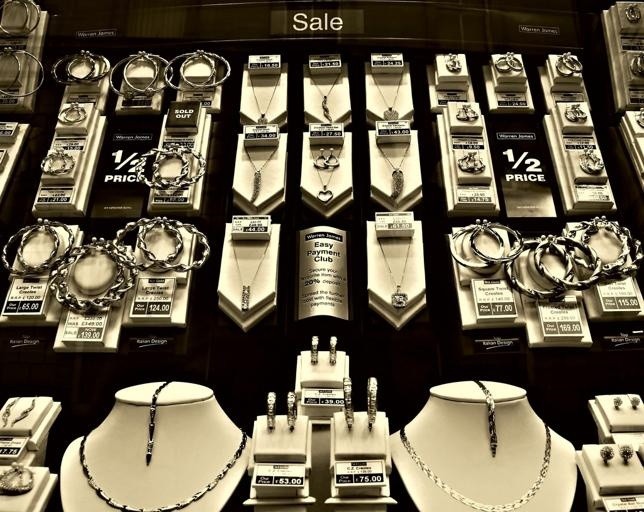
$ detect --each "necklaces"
[304,133,348,203]
[77,424,247,512]
[398,419,552,512]
[365,60,408,123]
[372,130,415,205]
[231,232,274,313]
[370,225,418,313]
[241,139,282,208]
[304,58,348,126]
[242,63,286,130]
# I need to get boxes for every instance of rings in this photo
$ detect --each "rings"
[631,395,640,411]
[601,444,616,466]
[612,396,623,409]
[618,444,633,463]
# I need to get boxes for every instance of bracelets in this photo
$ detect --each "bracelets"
[442,213,644,303]
[365,374,378,424]
[309,334,319,363]
[284,390,299,431]
[1,1,232,315]
[328,335,338,365]
[341,376,355,427]
[266,389,277,429]
